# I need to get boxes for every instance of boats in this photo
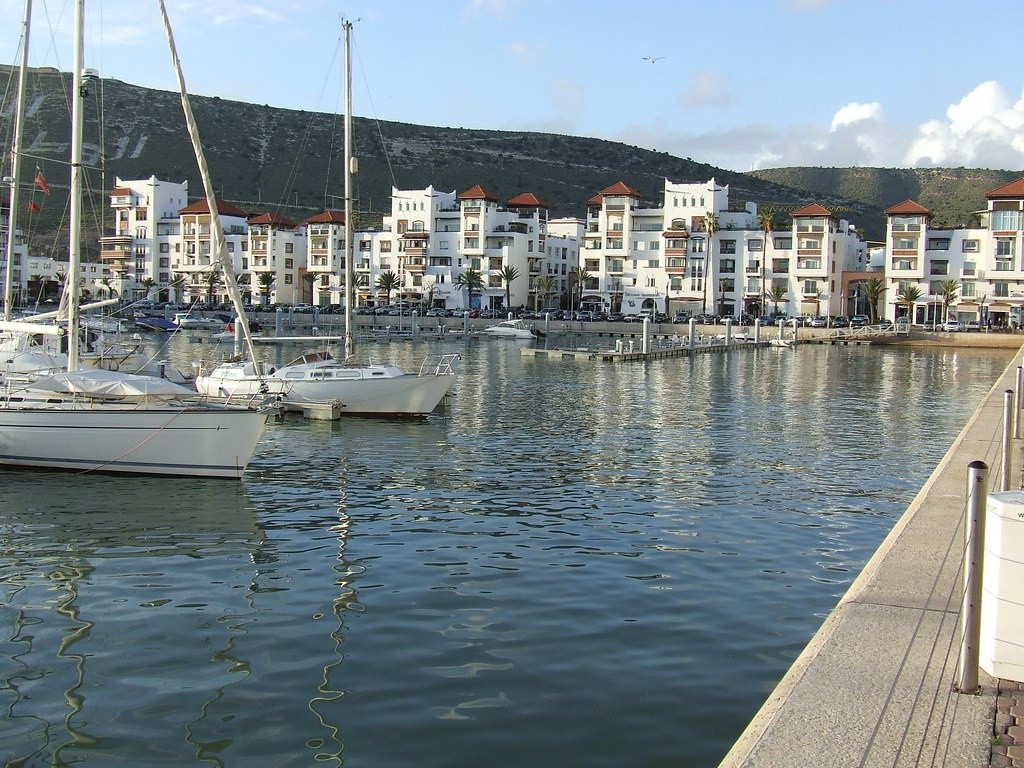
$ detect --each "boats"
[482,320,540,338]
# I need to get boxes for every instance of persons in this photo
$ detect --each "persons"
[1011,320,1024,330]
[988,318,992,330]
[998,318,1008,331]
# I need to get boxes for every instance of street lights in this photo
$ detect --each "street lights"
[652,287,659,323]
[289,284,297,304]
[571,286,576,321]
[740,290,745,325]
[420,285,426,316]
[492,285,497,318]
[533,286,537,312]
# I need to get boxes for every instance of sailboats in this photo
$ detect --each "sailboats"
[0,0,461,478]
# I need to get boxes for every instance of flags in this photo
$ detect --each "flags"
[35,172,50,196]
[29,201,40,212]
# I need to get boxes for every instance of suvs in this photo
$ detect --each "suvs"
[924,319,964,332]
[786,316,812,326]
[850,314,869,327]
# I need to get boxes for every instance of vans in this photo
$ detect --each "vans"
[636,308,660,322]
[134,300,154,307]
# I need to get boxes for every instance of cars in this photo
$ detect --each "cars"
[172,312,194,324]
[689,314,719,324]
[896,316,909,324]
[878,319,894,329]
[155,302,234,312]
[774,316,787,325]
[243,303,275,312]
[518,307,608,320]
[673,312,691,323]
[276,303,346,314]
[482,309,503,319]
[831,315,849,327]
[623,314,638,321]
[720,315,738,325]
[758,315,772,326]
[966,320,980,332]
[655,312,669,323]
[352,306,424,316]
[813,315,831,326]
[426,307,482,317]
[607,312,627,321]
